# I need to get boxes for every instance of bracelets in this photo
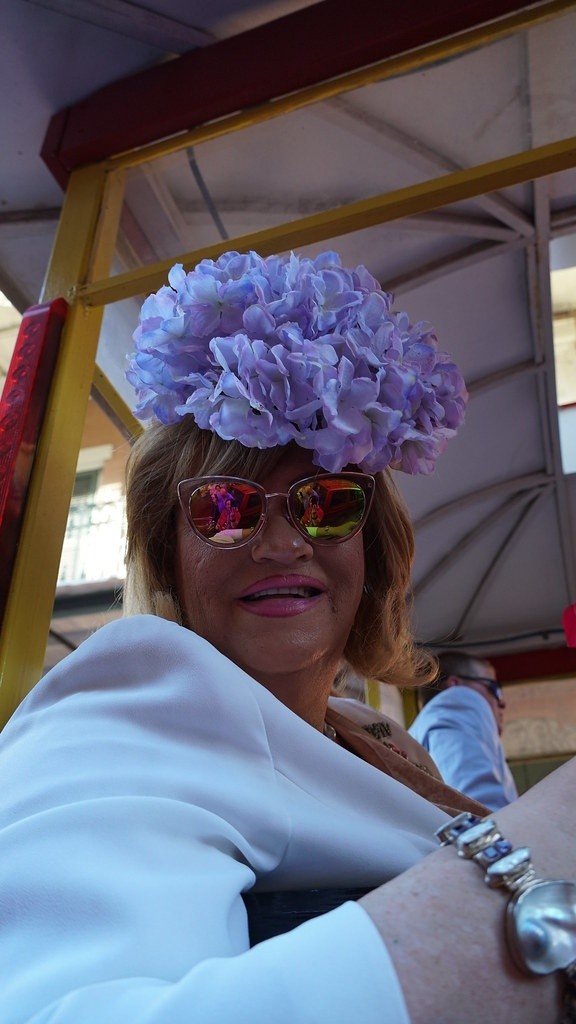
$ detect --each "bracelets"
[428,809,576,1014]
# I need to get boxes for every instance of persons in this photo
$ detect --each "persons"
[0,400,575,1024]
[402,649,522,810]
[203,481,357,547]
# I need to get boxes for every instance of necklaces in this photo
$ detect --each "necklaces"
[324,721,339,741]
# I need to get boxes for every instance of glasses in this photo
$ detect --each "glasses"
[175,472,375,551]
[441,671,506,697]
